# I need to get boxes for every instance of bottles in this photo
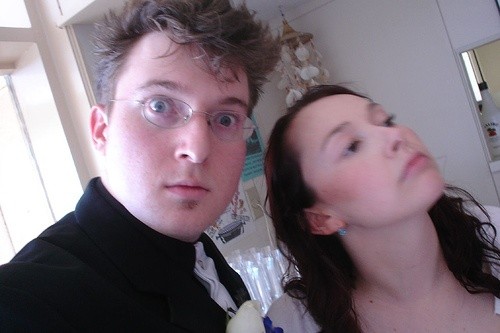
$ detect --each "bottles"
[477,82,500,158]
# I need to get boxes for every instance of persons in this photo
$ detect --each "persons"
[0,0,284,333]
[258,83,500,333]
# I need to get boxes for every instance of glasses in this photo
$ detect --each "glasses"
[108,93,257,143]
